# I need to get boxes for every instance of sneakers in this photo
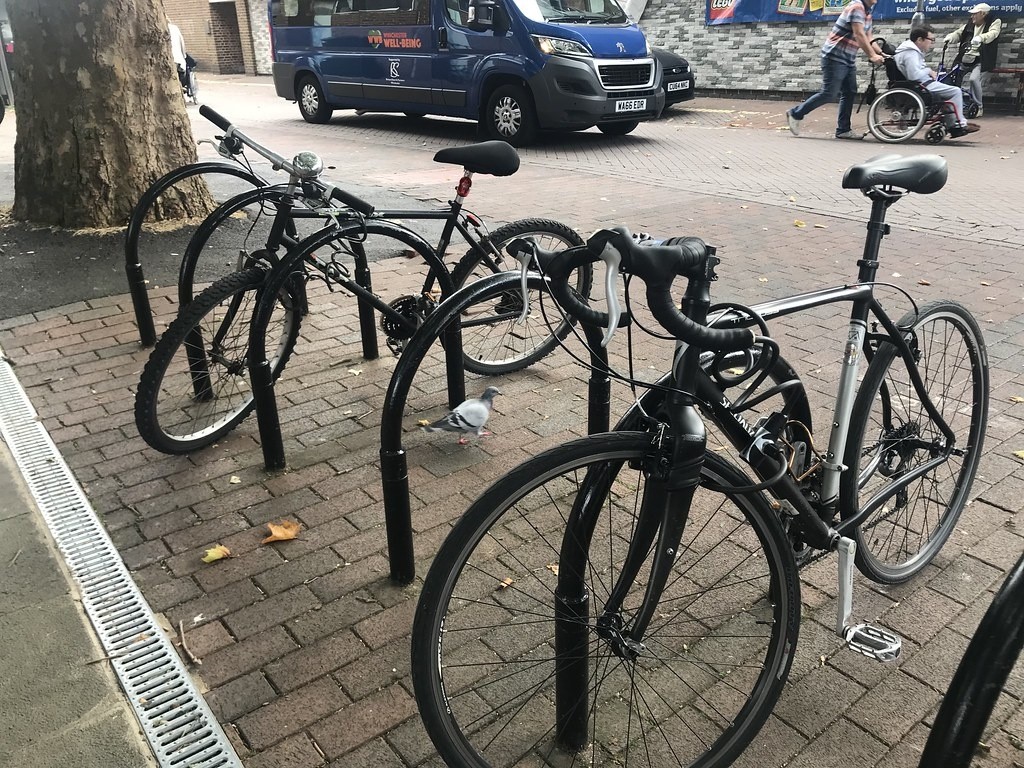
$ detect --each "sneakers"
[787,110,799,135]
[835,130,864,140]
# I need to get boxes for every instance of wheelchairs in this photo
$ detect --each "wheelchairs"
[177,56,198,105]
[871,38,978,145]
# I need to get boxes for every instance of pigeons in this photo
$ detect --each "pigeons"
[421,386,501,444]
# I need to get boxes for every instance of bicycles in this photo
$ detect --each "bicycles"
[410,152,990,768]
[133,103,593,456]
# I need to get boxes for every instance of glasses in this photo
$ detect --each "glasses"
[927,37,935,41]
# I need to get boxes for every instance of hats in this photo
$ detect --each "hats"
[968,3,990,13]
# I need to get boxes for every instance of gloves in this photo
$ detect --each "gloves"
[971,36,981,45]
[943,37,952,43]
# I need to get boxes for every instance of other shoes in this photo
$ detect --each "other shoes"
[967,123,981,128]
[976,108,984,117]
[951,126,979,137]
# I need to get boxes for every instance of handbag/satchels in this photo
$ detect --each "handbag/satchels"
[962,53,976,65]
[866,84,876,105]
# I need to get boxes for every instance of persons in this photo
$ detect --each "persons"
[892,24,981,137]
[942,3,1002,117]
[165,12,188,79]
[785,0,891,141]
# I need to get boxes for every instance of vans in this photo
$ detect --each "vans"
[268,1,664,145]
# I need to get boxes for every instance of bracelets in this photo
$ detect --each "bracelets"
[877,50,883,55]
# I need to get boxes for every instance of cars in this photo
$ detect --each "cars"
[646,44,696,117]
